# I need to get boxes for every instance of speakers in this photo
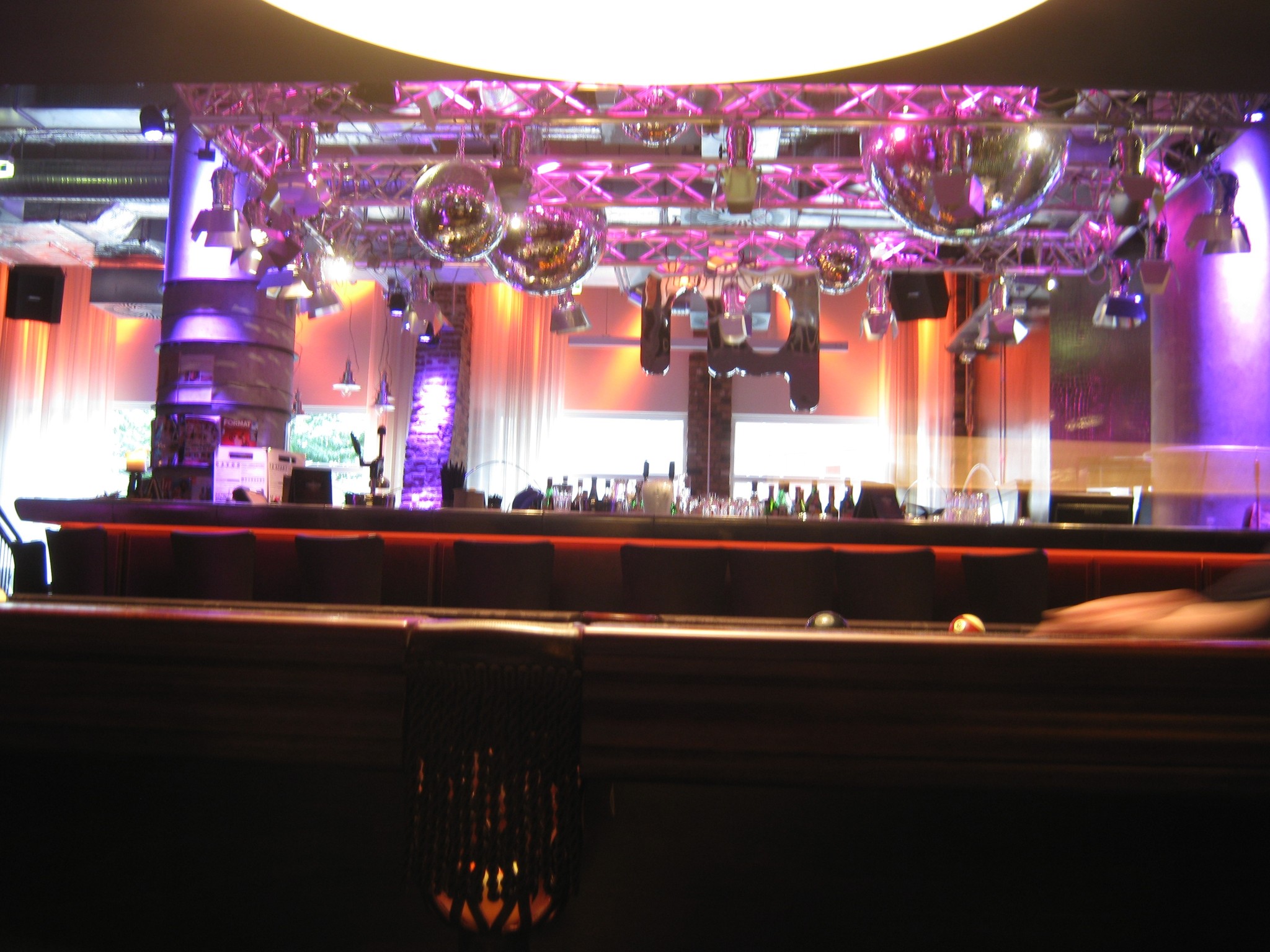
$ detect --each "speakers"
[885,272,950,321]
[4,263,64,324]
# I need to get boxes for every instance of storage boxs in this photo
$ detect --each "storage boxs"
[213,445,305,506]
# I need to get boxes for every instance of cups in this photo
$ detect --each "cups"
[552,485,573,512]
[944,491,991,524]
[641,479,673,516]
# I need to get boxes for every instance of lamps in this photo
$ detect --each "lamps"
[131,91,1251,356]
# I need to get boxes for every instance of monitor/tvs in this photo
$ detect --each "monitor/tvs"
[282,468,333,504]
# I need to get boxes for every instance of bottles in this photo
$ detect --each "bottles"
[668,461,854,521]
[541,462,649,514]
